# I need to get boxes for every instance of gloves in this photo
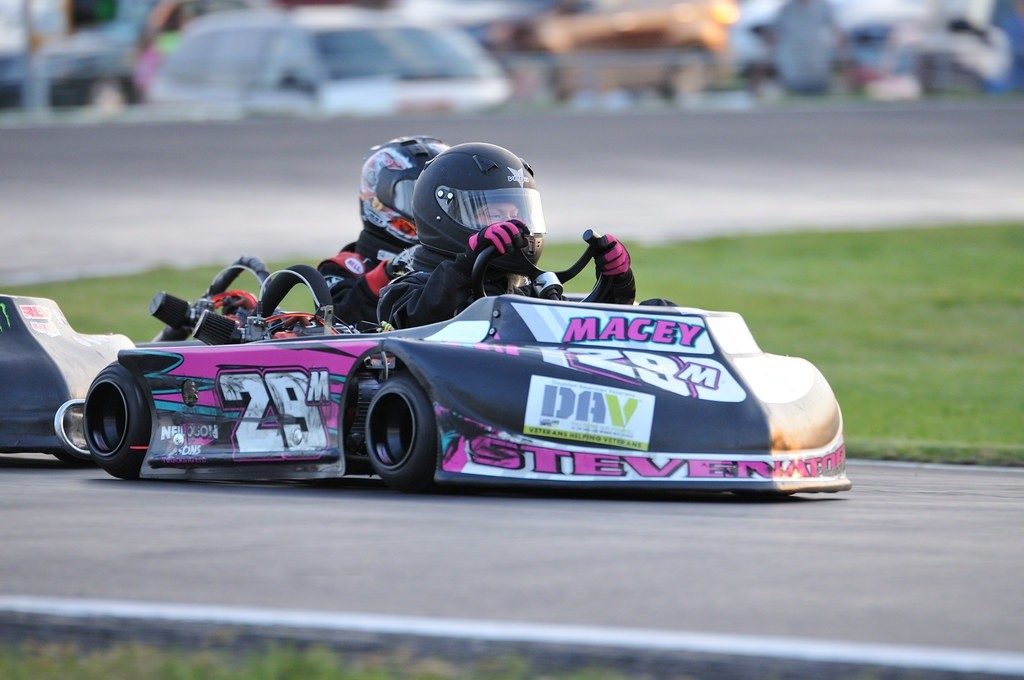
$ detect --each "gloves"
[591,234,632,286]
[386,245,421,279]
[466,218,530,255]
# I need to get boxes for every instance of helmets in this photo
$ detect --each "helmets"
[359,136,451,254]
[411,142,547,284]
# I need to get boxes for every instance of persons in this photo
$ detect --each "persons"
[317,130,452,328]
[373,141,635,324]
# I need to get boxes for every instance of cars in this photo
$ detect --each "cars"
[0,0,1023,119]
